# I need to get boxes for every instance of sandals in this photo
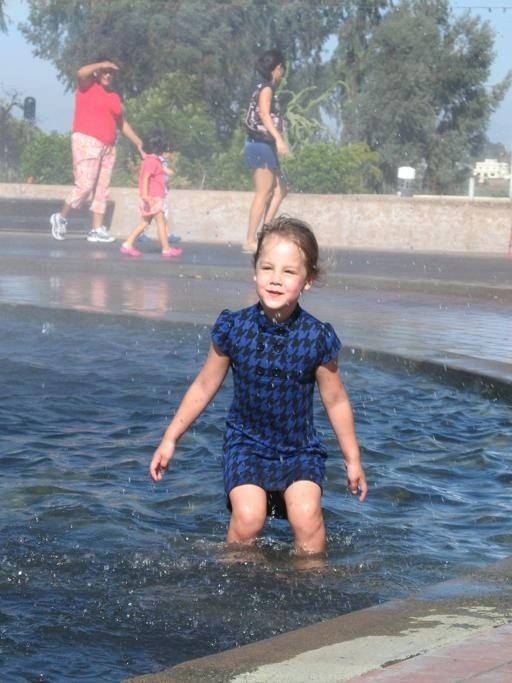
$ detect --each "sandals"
[160,247,183,259]
[119,244,141,257]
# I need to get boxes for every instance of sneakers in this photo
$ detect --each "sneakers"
[50,213,68,240]
[86,225,115,242]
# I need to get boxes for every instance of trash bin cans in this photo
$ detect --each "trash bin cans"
[396,165,416,197]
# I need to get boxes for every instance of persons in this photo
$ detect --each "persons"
[119,131,184,259]
[50,57,143,243]
[242,52,289,252]
[150,216,367,554]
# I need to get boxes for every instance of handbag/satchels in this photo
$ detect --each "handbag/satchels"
[244,99,284,141]
